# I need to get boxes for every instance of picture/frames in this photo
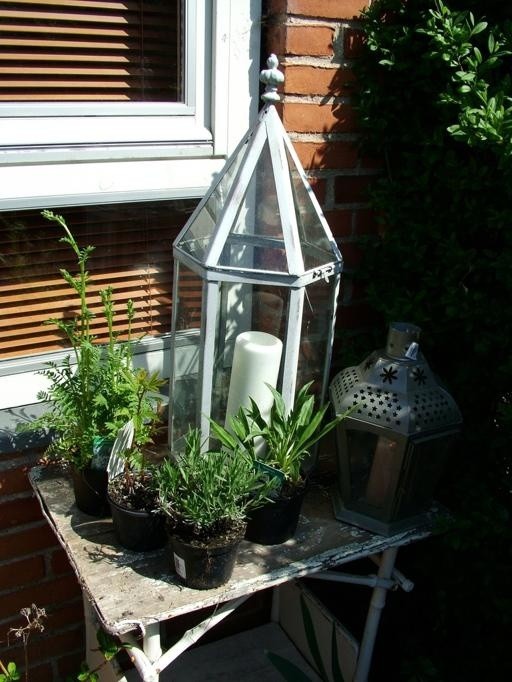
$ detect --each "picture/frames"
[28,457,434,682]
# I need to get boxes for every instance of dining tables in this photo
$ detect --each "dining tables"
[206,381,359,545]
[38,206,156,522]
[156,425,256,590]
[107,369,172,551]
